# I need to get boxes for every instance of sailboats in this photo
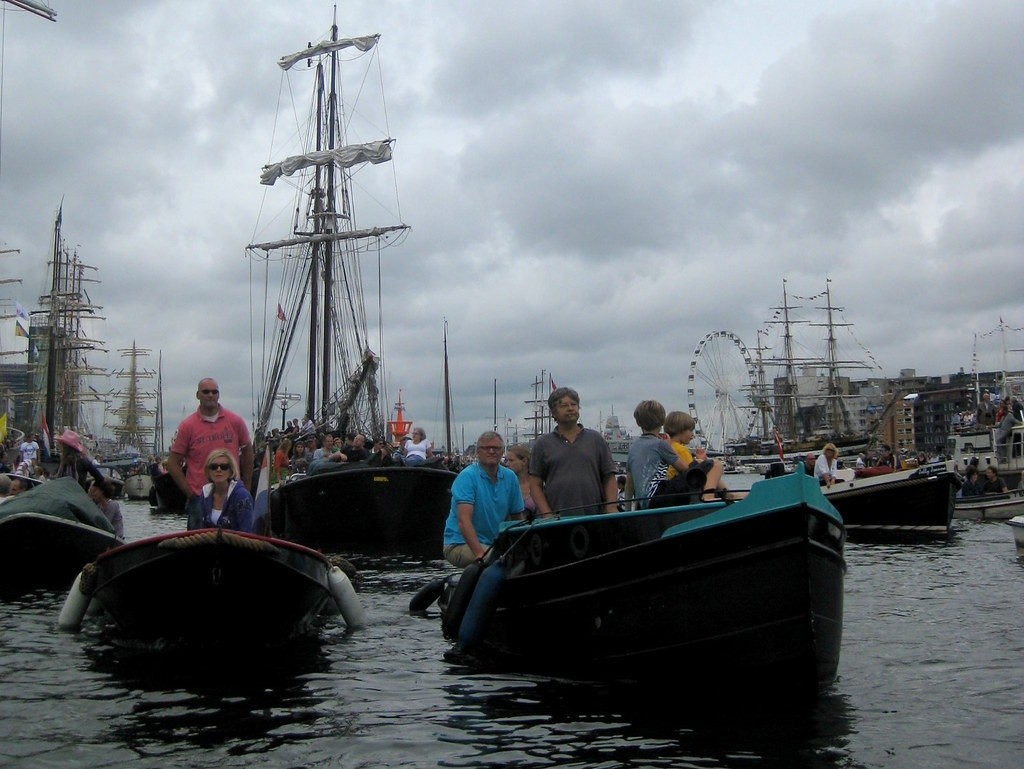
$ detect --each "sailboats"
[726,275,903,470]
[947,319,1024,493]
[241,2,462,554]
[0,192,170,502]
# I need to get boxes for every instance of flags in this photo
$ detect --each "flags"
[15,300,29,321]
[0,411,7,444]
[773,430,783,459]
[41,412,50,460]
[15,320,29,338]
[278,303,287,321]
[30,341,40,359]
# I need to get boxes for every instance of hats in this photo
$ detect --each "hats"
[54,430,85,453]
[1011,396,1019,401]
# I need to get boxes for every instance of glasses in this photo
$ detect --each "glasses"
[200,389,219,394]
[207,463,230,471]
[479,446,503,451]
[826,448,835,452]
[376,443,383,445]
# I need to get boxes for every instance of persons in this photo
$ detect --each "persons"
[266,414,433,494]
[166,377,254,531]
[856,393,1024,497]
[625,400,723,512]
[528,387,619,519]
[443,431,525,569]
[199,448,254,534]
[663,411,750,499]
[617,476,626,512]
[814,443,838,488]
[804,453,816,477]
[0,430,169,539]
[506,447,536,513]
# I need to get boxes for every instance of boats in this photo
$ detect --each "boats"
[1003,514,1024,560]
[0,469,124,580]
[954,487,1024,520]
[91,525,359,665]
[437,460,848,707]
[821,460,967,538]
